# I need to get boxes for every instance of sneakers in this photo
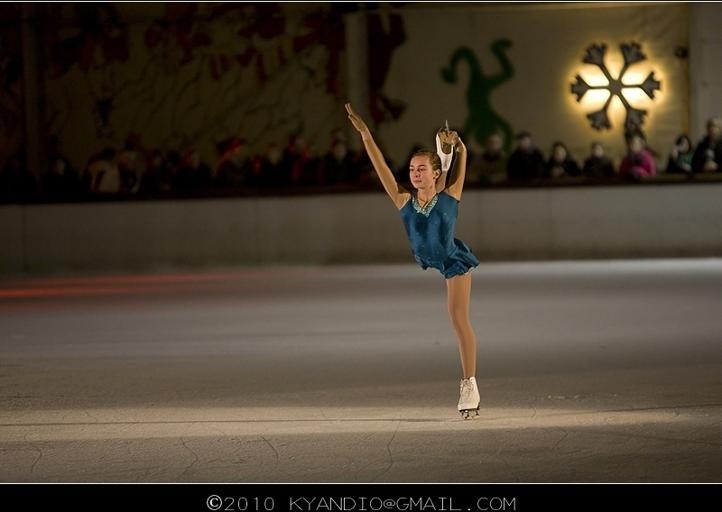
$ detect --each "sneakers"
[435,128,454,171]
[457,377,480,411]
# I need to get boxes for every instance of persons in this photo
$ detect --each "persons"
[344,102,480,412]
[1,116,722,193]
